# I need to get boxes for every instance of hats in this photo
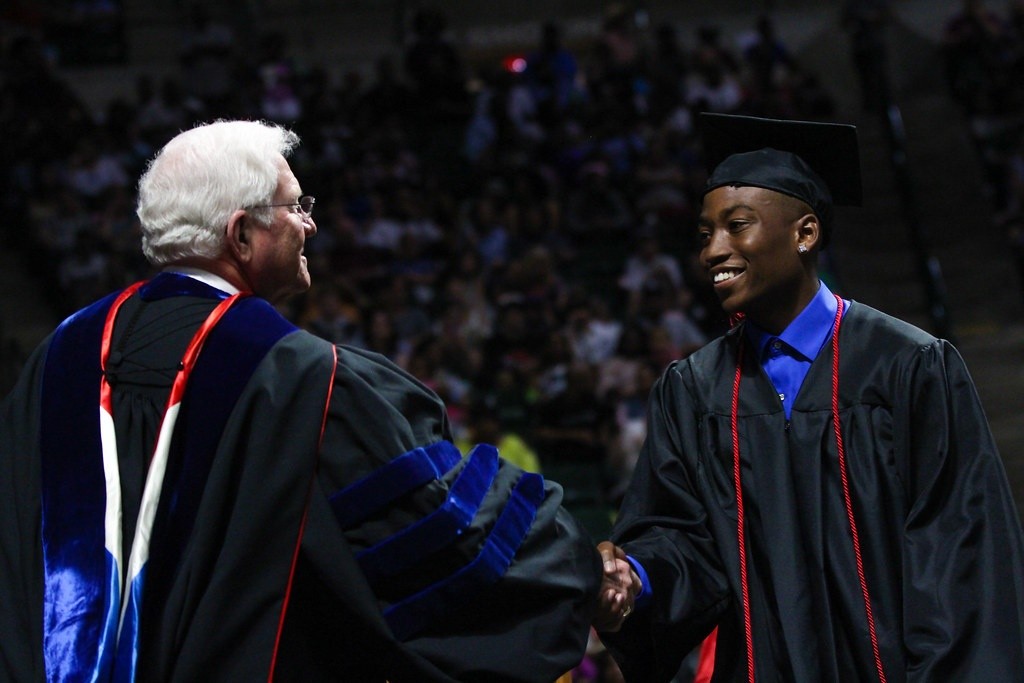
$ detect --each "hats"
[698,111,861,251]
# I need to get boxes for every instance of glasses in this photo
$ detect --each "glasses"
[243,196,316,218]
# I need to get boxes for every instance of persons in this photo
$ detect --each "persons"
[0,1,1024,683]
